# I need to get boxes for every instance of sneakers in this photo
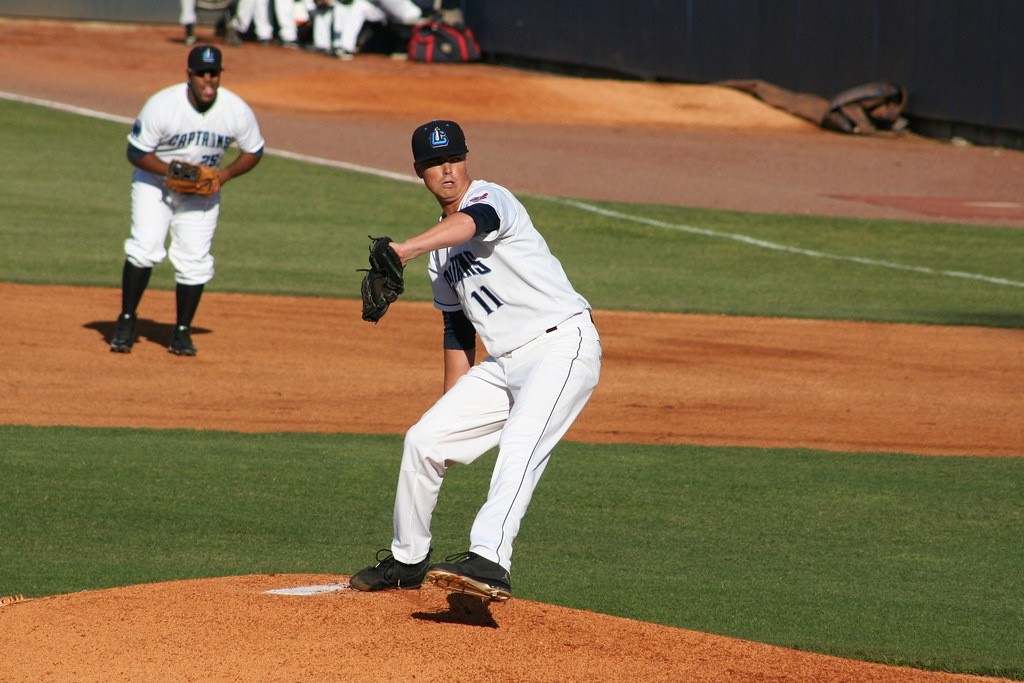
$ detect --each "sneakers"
[425,551,512,603]
[110,313,137,353]
[167,322,196,356]
[349,547,433,592]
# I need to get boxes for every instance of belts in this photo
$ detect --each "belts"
[546,312,594,333]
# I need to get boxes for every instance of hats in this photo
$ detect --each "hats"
[411,119,469,164]
[188,45,222,71]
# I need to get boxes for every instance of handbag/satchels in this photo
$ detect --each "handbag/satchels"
[407,21,481,63]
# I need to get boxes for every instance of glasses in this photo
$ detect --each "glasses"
[190,71,219,77]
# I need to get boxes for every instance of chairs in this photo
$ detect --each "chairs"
[195,0,239,44]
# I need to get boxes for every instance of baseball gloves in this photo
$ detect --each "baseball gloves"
[162,156,221,198]
[354,231,405,324]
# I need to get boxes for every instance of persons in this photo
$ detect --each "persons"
[350,119,603,600]
[179,0,428,61]
[111,44,264,357]
